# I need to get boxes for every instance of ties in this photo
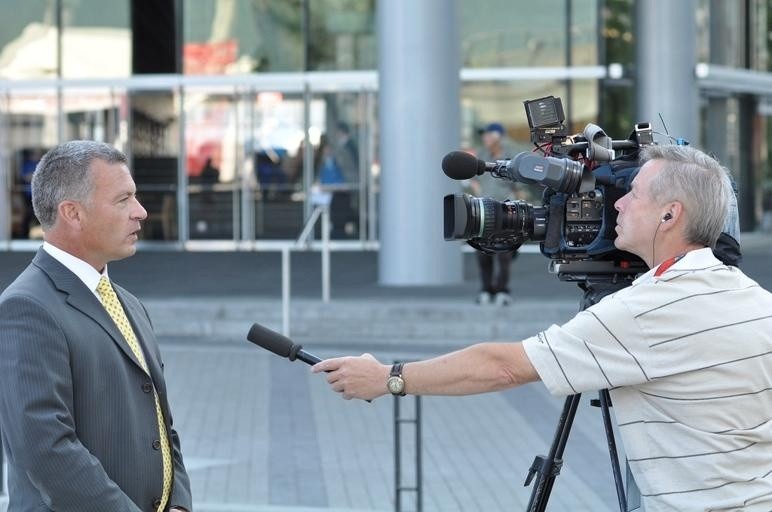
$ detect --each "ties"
[96,275,173,511]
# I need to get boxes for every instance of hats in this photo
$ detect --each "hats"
[476,124,504,134]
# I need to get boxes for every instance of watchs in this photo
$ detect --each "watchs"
[386,359,409,399]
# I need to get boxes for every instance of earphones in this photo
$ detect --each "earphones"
[661,213,671,223]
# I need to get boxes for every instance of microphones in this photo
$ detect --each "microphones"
[246,323,374,403]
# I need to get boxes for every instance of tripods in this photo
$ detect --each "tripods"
[523,291,628,512]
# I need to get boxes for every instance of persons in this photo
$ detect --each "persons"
[307,140,771,512]
[1,135,195,512]
[15,146,38,238]
[198,156,222,185]
[313,120,364,210]
[461,121,523,308]
